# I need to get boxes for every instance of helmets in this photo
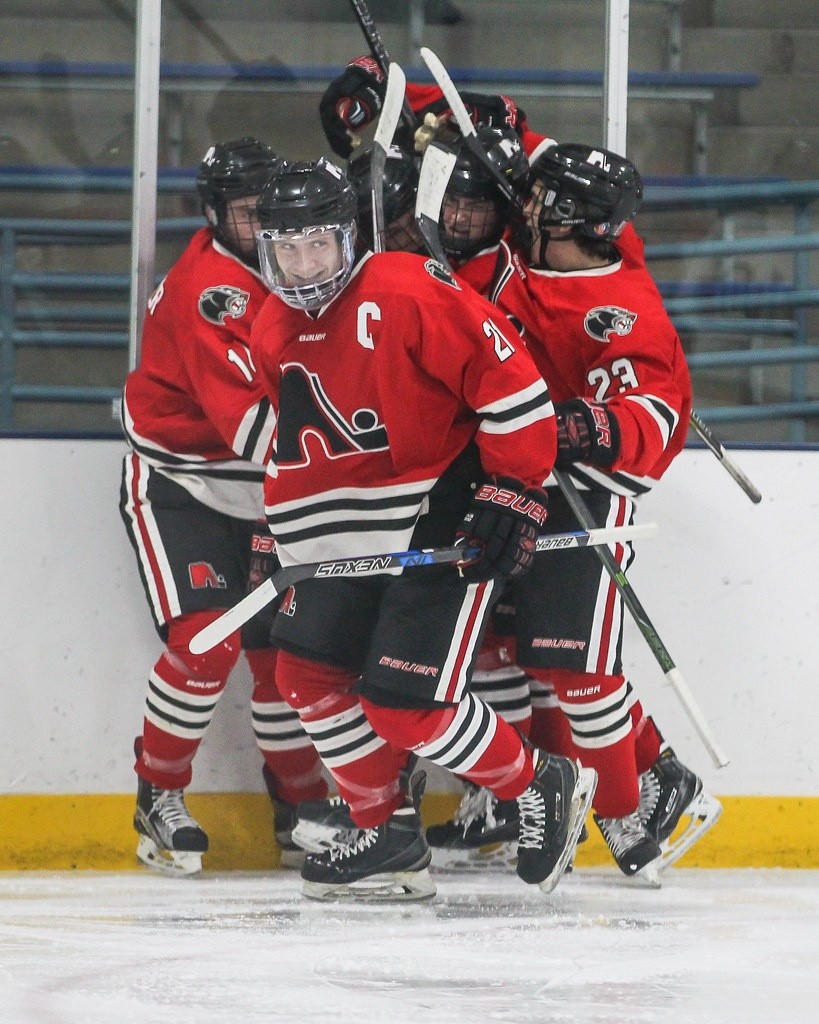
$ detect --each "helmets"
[349,143,430,255]
[431,122,530,258]
[509,142,644,240]
[196,135,277,266]
[254,156,359,310]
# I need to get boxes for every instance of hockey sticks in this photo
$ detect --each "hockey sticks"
[415,140,731,770]
[368,61,407,252]
[187,523,644,657]
[350,1,418,135]
[421,43,763,507]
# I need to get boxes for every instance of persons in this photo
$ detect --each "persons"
[248,161,599,903]
[119,55,721,885]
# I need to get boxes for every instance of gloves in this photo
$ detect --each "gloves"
[413,87,526,150]
[319,56,388,160]
[450,471,550,583]
[244,523,280,593]
[552,394,618,469]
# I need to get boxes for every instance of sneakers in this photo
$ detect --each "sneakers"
[592,812,662,888]
[515,747,598,895]
[299,797,436,905]
[131,735,207,876]
[424,785,518,872]
[638,745,722,871]
[290,796,359,854]
[262,763,308,868]
